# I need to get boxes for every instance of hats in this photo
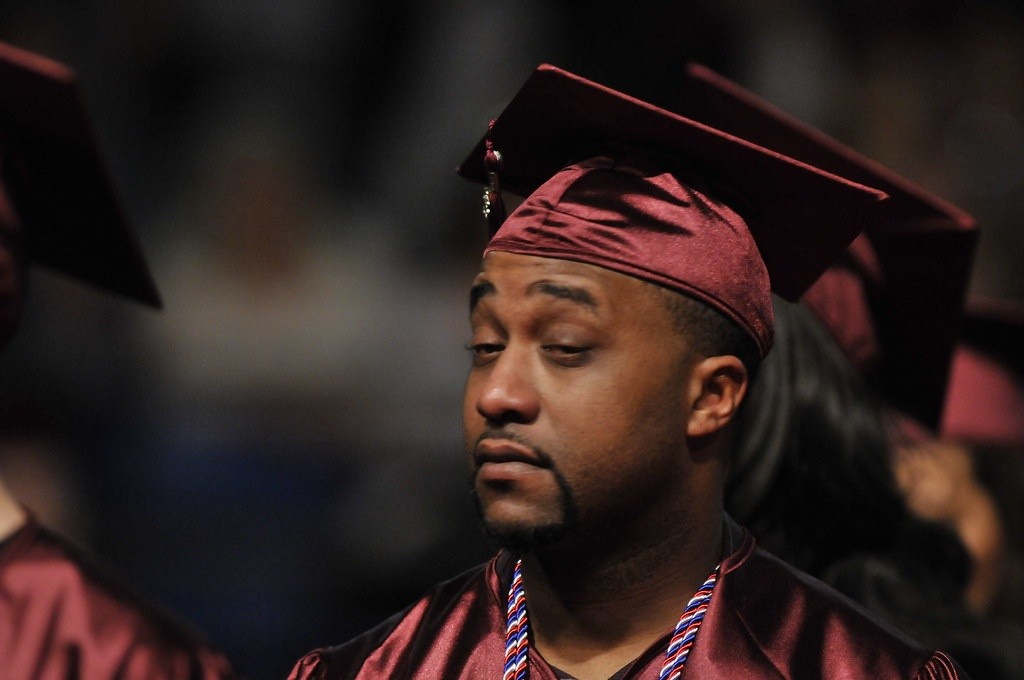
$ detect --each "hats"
[888,299,1024,447]
[0,42,162,307]
[669,61,985,435]
[456,63,892,364]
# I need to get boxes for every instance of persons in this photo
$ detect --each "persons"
[288,62,1024,680]
[0,45,230,680]
